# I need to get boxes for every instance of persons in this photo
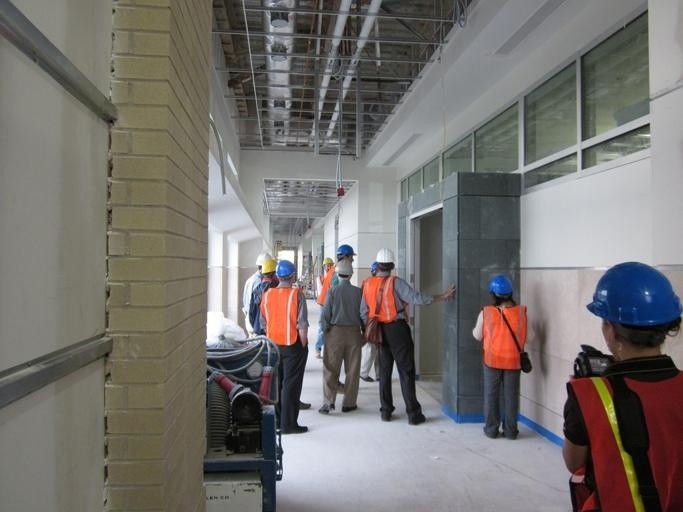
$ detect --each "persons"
[313,260,363,415]
[359,247,457,425]
[356,259,383,382]
[259,258,310,435]
[314,244,357,356]
[316,255,338,306]
[472,274,527,438]
[242,252,270,338]
[560,261,683,512]
[248,257,277,341]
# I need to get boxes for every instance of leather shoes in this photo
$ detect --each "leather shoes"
[318,403,335,414]
[360,376,374,382]
[380,410,392,422]
[282,425,309,435]
[298,401,312,411]
[407,414,426,425]
[341,404,359,413]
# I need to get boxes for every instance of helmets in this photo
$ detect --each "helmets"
[322,257,334,266]
[335,260,353,276]
[487,275,513,299]
[370,261,378,274]
[275,260,297,278]
[336,244,356,255]
[375,248,394,263]
[585,261,683,331]
[261,259,277,274]
[255,252,275,266]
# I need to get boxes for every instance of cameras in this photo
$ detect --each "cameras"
[574,344,614,378]
